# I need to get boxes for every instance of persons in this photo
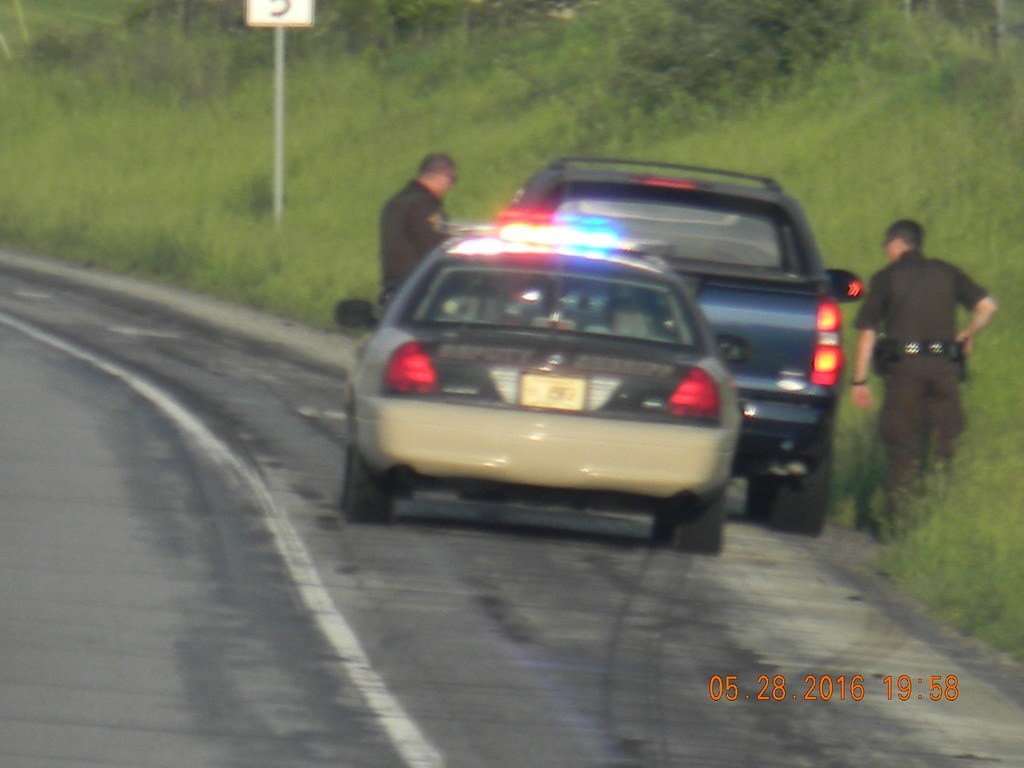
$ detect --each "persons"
[379,152,457,307]
[850,216,997,531]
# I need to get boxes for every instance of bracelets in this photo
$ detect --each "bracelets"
[851,381,867,386]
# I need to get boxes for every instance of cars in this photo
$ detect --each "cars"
[336,209,740,554]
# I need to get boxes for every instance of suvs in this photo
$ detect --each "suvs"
[514,159,867,541]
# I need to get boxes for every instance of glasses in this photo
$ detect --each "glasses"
[884,238,895,247]
[437,170,456,185]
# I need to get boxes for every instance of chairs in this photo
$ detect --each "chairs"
[611,307,648,338]
[438,291,499,323]
